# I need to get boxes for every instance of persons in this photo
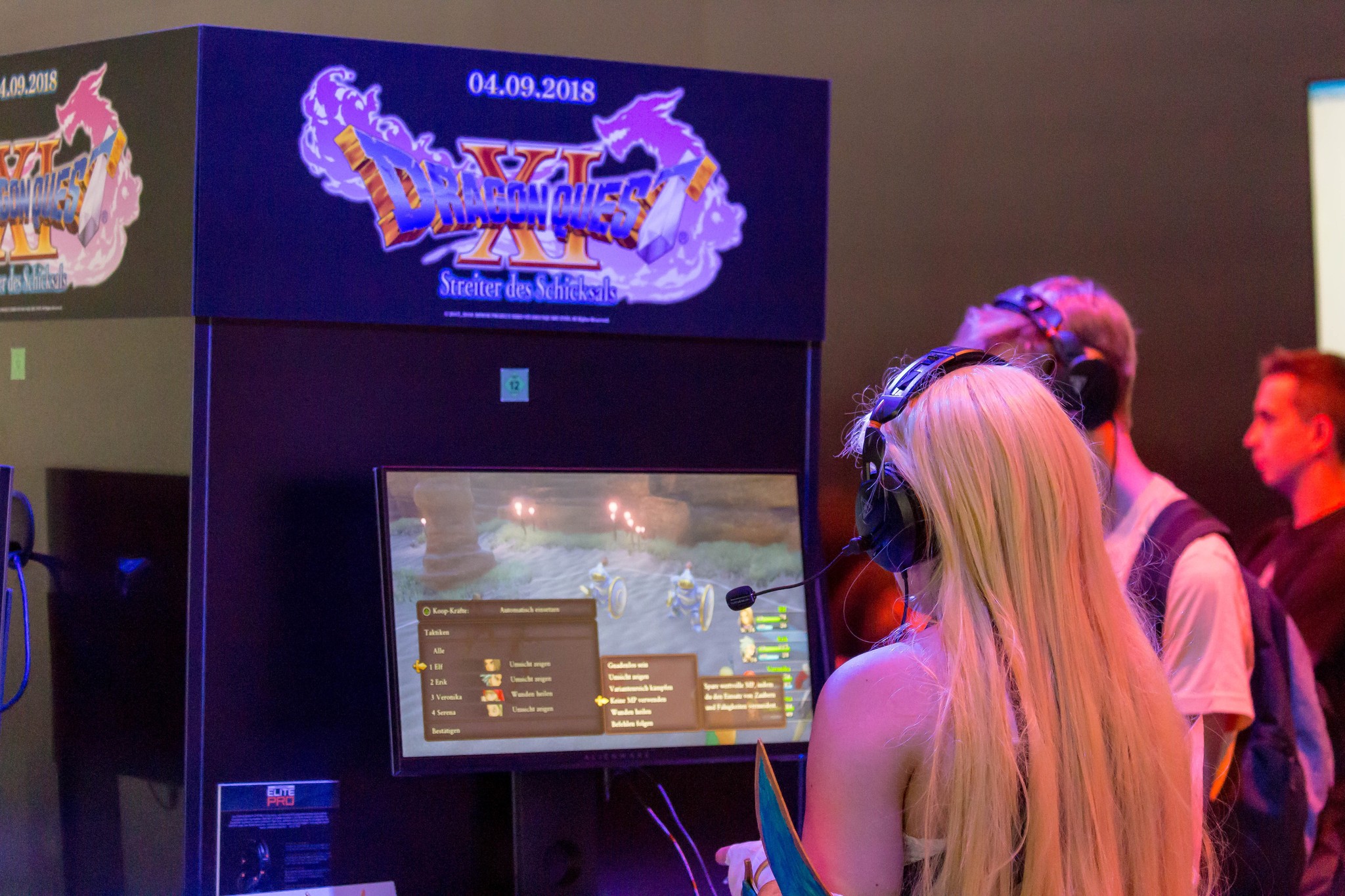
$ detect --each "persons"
[714,345,1202,896]
[954,279,1254,896]
[1237,347,1345,778]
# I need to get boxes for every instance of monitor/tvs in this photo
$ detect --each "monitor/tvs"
[369,465,832,779]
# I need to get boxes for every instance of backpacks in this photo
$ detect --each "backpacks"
[1122,501,1336,896]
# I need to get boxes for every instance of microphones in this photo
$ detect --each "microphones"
[726,536,871,611]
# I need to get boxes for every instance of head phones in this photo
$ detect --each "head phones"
[992,285,1119,431]
[855,346,1013,573]
